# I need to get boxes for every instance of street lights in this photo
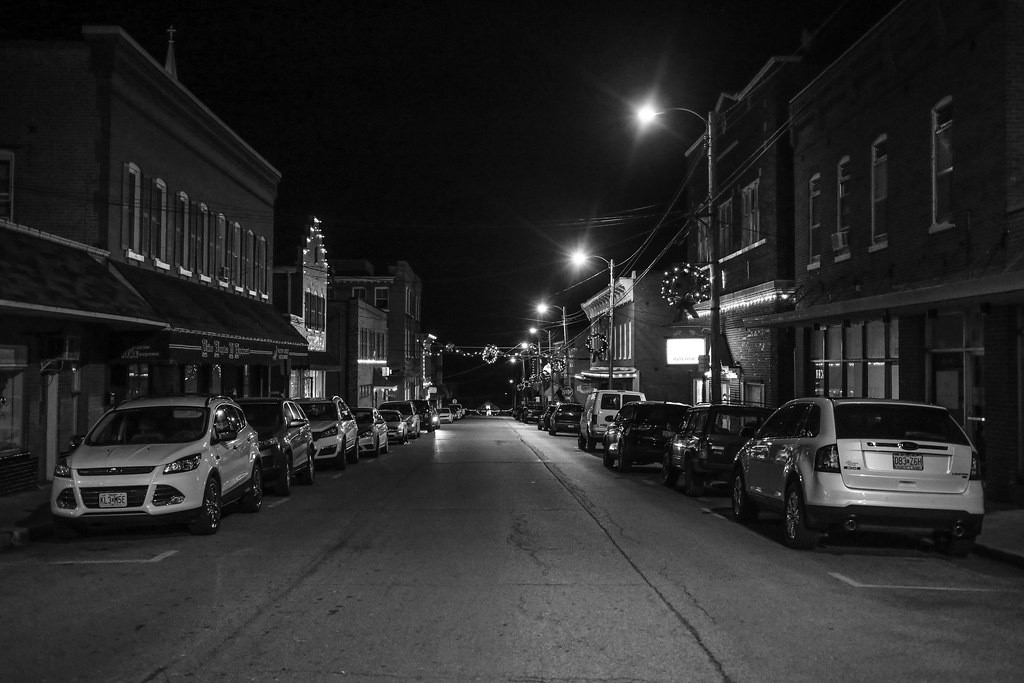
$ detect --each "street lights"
[522,342,539,379]
[531,328,552,351]
[637,106,722,403]
[510,380,516,411]
[510,358,525,384]
[572,255,614,391]
[539,306,568,346]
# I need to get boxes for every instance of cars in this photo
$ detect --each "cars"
[604,401,693,474]
[538,404,556,430]
[550,403,582,435]
[733,396,986,553]
[378,410,408,445]
[350,407,389,456]
[436,408,454,424]
[664,404,776,496]
[378,401,421,439]
[455,408,462,419]
[451,411,457,421]
[431,406,440,429]
[288,396,360,466]
[406,400,433,433]
[234,398,315,496]
[50,395,263,535]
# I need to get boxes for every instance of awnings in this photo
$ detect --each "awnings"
[0,226,170,337]
[105,258,310,367]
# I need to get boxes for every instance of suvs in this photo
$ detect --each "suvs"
[523,402,544,424]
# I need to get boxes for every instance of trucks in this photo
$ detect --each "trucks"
[578,391,646,452]
